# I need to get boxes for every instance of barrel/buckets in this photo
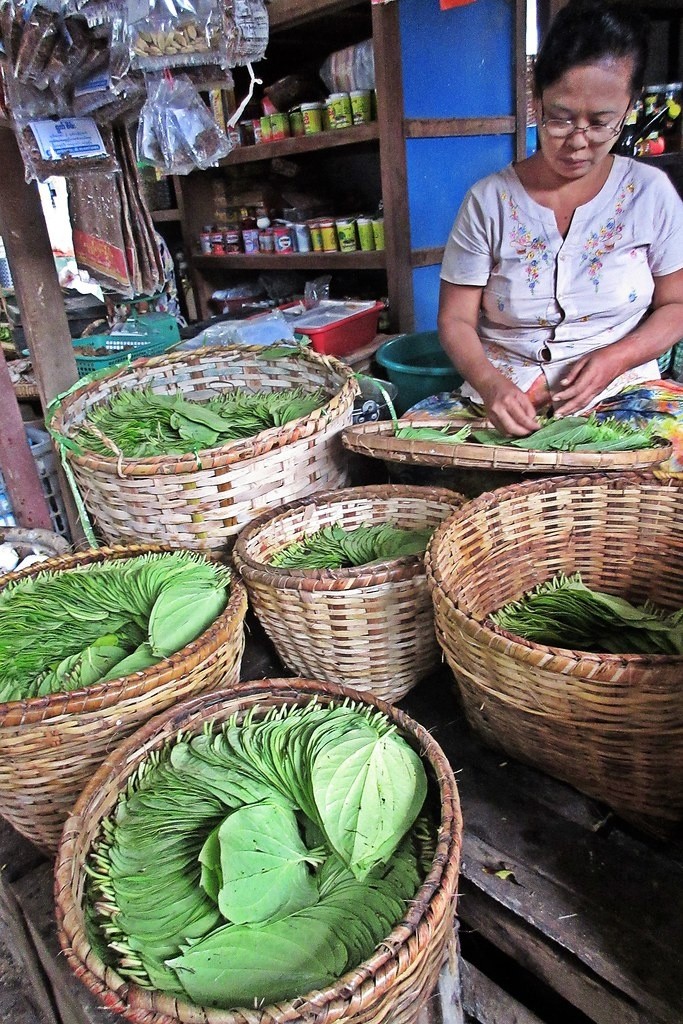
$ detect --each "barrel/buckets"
[351,330,464,425]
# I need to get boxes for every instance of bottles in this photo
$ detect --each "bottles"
[607,82,683,158]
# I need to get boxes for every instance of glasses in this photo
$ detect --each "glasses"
[540,89,633,144]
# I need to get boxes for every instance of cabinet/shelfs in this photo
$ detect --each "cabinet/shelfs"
[151,0,528,337]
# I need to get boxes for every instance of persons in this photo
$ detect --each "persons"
[400,0,683,439]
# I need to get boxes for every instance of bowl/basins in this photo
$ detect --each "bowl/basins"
[208,293,264,314]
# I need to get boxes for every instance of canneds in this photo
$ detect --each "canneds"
[200,223,310,255]
[241,106,304,145]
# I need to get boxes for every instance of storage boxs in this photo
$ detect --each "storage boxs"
[247,298,384,359]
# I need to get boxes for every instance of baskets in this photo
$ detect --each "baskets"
[421,469,683,818]
[0,546,248,860]
[22,334,166,379]
[232,481,470,705]
[44,342,363,572]
[340,420,674,499]
[54,676,463,1024]
